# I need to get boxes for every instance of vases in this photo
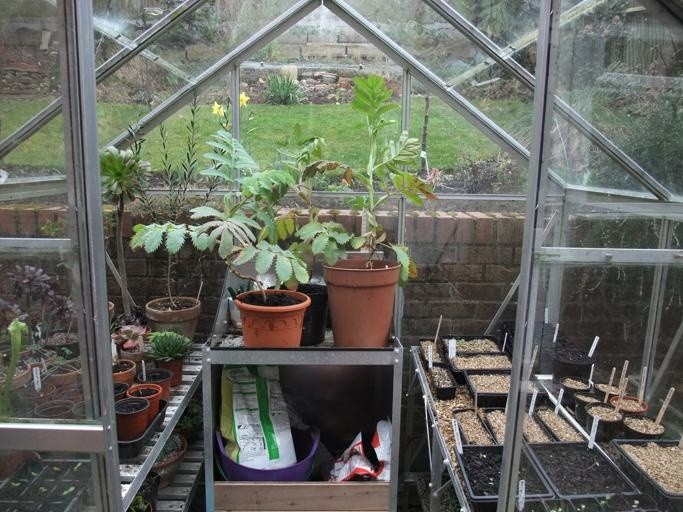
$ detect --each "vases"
[416,320,683,512]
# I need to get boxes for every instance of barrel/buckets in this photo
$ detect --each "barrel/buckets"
[216,421,322,483]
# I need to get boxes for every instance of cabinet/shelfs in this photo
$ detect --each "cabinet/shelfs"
[2,346,197,510]
[201,251,403,512]
[400,346,594,512]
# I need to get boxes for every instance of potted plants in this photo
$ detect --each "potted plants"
[115,380,129,398]
[130,473,158,511]
[128,383,161,421]
[1,300,113,508]
[309,73,442,347]
[112,359,137,383]
[149,333,190,386]
[138,369,171,399]
[266,282,328,349]
[115,397,148,441]
[139,92,227,341]
[143,297,198,339]
[211,137,311,349]
[152,430,186,486]
